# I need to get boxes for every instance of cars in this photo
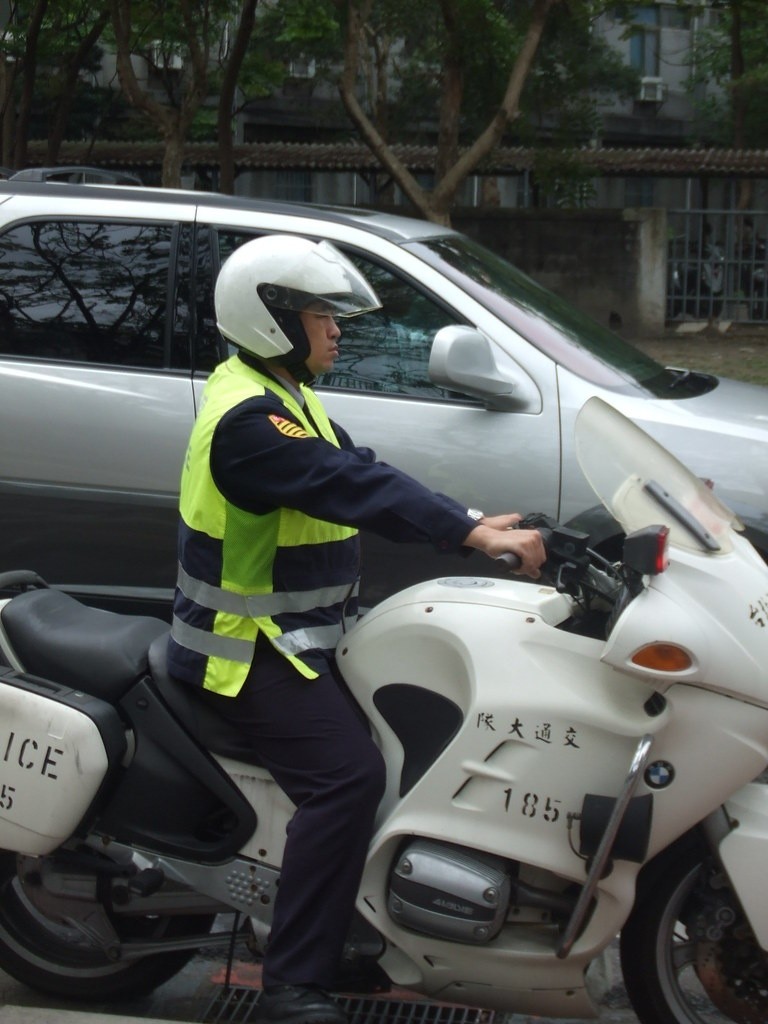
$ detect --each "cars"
[0,166,766,610]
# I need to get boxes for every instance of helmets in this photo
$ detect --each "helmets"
[213,234,383,369]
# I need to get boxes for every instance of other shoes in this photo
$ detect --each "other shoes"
[252,989,349,1024]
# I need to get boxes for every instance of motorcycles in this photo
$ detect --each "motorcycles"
[0,393,767,1024]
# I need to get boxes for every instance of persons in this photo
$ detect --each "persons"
[166,233,546,1024]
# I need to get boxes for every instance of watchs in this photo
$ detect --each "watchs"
[468,509,484,522]
